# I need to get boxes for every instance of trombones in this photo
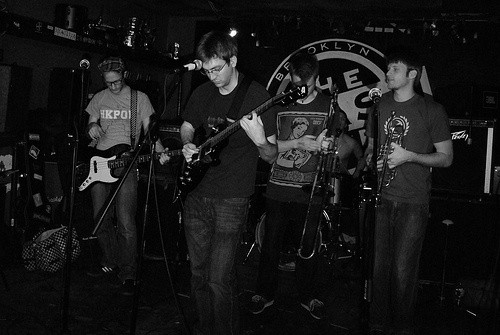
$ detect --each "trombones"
[296,83,340,259]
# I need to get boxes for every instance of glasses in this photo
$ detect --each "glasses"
[200,62,227,76]
[106,79,122,86]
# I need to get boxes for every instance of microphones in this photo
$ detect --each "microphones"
[78,58,91,70]
[368,88,383,103]
[183,59,203,72]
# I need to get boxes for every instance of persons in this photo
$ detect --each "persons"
[84,57,171,305]
[248,48,343,313]
[364,52,454,335]
[178,29,278,335]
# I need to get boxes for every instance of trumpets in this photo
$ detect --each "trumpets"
[362,111,403,208]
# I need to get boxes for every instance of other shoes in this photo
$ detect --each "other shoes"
[122,280,135,295]
[95,264,116,273]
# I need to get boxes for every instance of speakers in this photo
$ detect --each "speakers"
[416,195,500,278]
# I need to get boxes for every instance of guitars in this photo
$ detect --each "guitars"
[171,84,309,208]
[74,143,183,191]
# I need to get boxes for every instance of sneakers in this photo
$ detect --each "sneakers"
[248,294,275,314]
[302,299,326,319]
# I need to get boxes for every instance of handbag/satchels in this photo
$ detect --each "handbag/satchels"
[20,226,81,274]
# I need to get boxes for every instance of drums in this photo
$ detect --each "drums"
[255,209,332,272]
